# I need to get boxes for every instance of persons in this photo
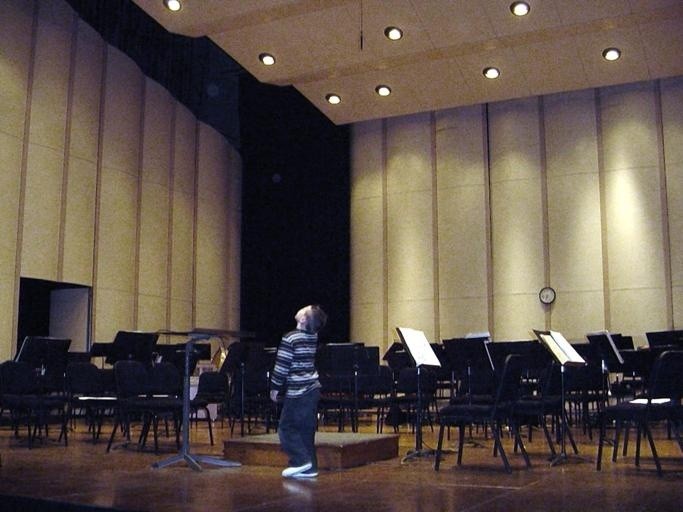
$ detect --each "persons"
[267,305,327,479]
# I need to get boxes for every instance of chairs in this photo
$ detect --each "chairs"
[360,351,683,476]
[0,355,231,456]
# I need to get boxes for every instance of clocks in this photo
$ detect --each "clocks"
[539,287,556,305]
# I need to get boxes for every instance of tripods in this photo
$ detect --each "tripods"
[550,370,596,468]
[403,364,438,464]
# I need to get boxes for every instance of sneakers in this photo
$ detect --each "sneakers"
[282,462,319,478]
